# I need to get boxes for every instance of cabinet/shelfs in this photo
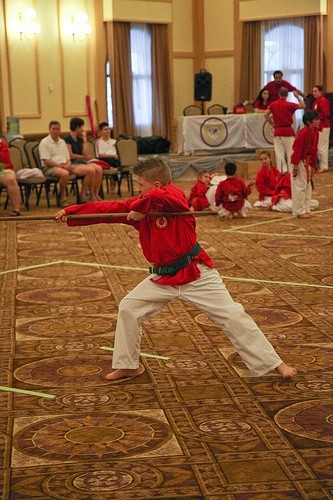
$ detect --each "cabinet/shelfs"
[304,92,333,150]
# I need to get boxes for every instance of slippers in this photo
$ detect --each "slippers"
[10,210,21,217]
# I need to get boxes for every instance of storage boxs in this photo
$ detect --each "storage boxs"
[255,148,276,167]
[233,104,247,114]
[234,159,263,181]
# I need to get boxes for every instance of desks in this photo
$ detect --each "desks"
[175,112,298,156]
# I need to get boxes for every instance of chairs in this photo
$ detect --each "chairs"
[207,103,224,115]
[0,134,139,212]
[183,104,203,116]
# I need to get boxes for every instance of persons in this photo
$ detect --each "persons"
[187,71,331,217]
[0,137,21,215]
[95,122,118,158]
[63,117,103,201]
[54,156,296,380]
[39,121,95,207]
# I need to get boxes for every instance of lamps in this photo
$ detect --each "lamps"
[17,7,41,41]
[70,10,92,43]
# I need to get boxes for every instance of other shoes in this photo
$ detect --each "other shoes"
[60,199,70,208]
[90,197,102,203]
[79,199,90,204]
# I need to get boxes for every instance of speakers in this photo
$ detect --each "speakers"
[195,72,212,100]
